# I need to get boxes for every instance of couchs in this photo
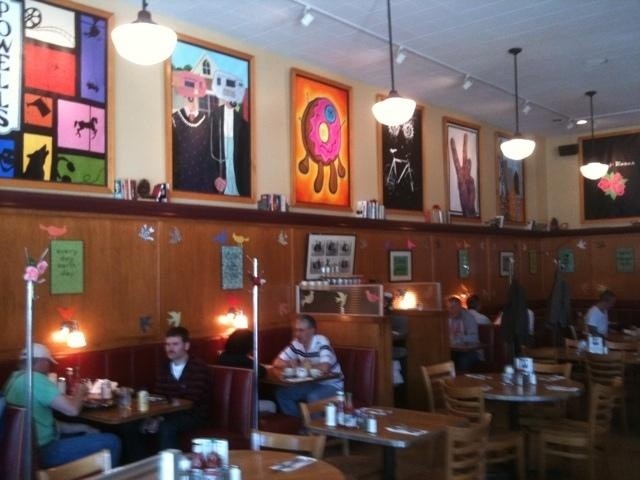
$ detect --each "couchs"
[254,404,300,435]
[147,366,254,451]
[1,406,29,479]
[331,347,377,408]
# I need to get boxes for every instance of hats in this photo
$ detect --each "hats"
[19,342,59,366]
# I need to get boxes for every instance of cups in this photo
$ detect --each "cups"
[312,369,319,377]
[118,388,133,416]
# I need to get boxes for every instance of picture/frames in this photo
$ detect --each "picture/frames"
[220,245,243,290]
[388,249,412,282]
[442,117,481,217]
[377,95,428,215]
[529,250,537,273]
[556,249,575,273]
[305,233,356,282]
[164,34,257,204]
[1,2,116,193]
[289,68,354,212]
[616,248,634,273]
[49,240,85,296]
[578,132,640,225]
[458,249,471,280]
[498,251,514,278]
[495,132,526,226]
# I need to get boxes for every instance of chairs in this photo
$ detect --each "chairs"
[249,431,332,460]
[421,360,456,413]
[442,414,492,478]
[603,334,640,351]
[582,351,631,434]
[531,361,571,379]
[536,379,622,479]
[563,337,580,362]
[439,382,525,479]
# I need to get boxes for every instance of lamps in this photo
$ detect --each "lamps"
[371,0,417,126]
[500,47,536,161]
[580,91,610,181]
[48,321,87,349]
[390,291,423,310]
[215,310,247,337]
[108,1,176,68]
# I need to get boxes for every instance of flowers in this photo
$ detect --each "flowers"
[598,162,629,200]
[23,246,49,282]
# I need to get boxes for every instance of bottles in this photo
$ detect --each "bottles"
[206,439,224,480]
[138,390,149,412]
[344,392,357,429]
[337,390,345,426]
[189,445,205,480]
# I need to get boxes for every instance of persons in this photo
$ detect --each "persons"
[444,132,477,218]
[216,328,277,414]
[3,341,122,471]
[274,313,345,420]
[494,280,535,345]
[583,288,617,344]
[137,328,211,449]
[443,297,484,375]
[465,293,492,328]
